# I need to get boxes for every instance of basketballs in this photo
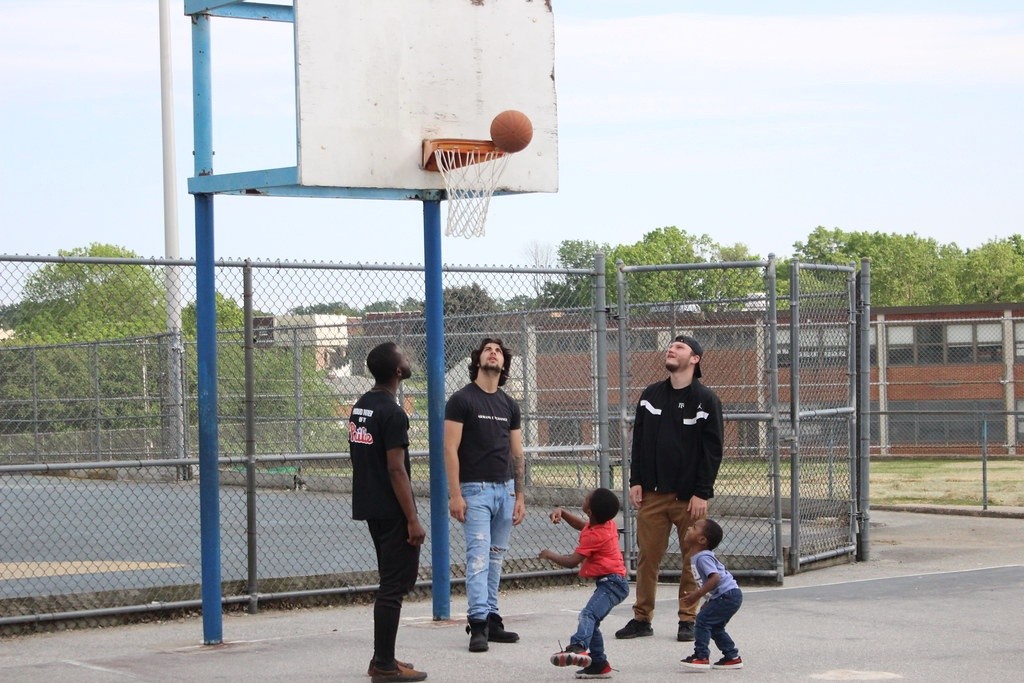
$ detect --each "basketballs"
[490,110,533,154]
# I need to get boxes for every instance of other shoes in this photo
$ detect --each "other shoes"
[368,658,414,676]
[370,663,427,683]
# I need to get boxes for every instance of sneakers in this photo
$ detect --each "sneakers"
[615,618,654,639]
[679,654,710,669]
[549,640,592,668]
[677,620,695,641]
[712,655,743,669]
[573,660,621,679]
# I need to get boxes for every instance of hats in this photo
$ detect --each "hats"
[675,335,703,379]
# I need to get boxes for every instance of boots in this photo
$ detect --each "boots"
[465,615,490,652]
[488,611,519,643]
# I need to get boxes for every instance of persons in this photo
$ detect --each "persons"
[348,342,428,683]
[614,334,724,639]
[537,487,630,678]
[442,337,526,652]
[680,519,744,669]
